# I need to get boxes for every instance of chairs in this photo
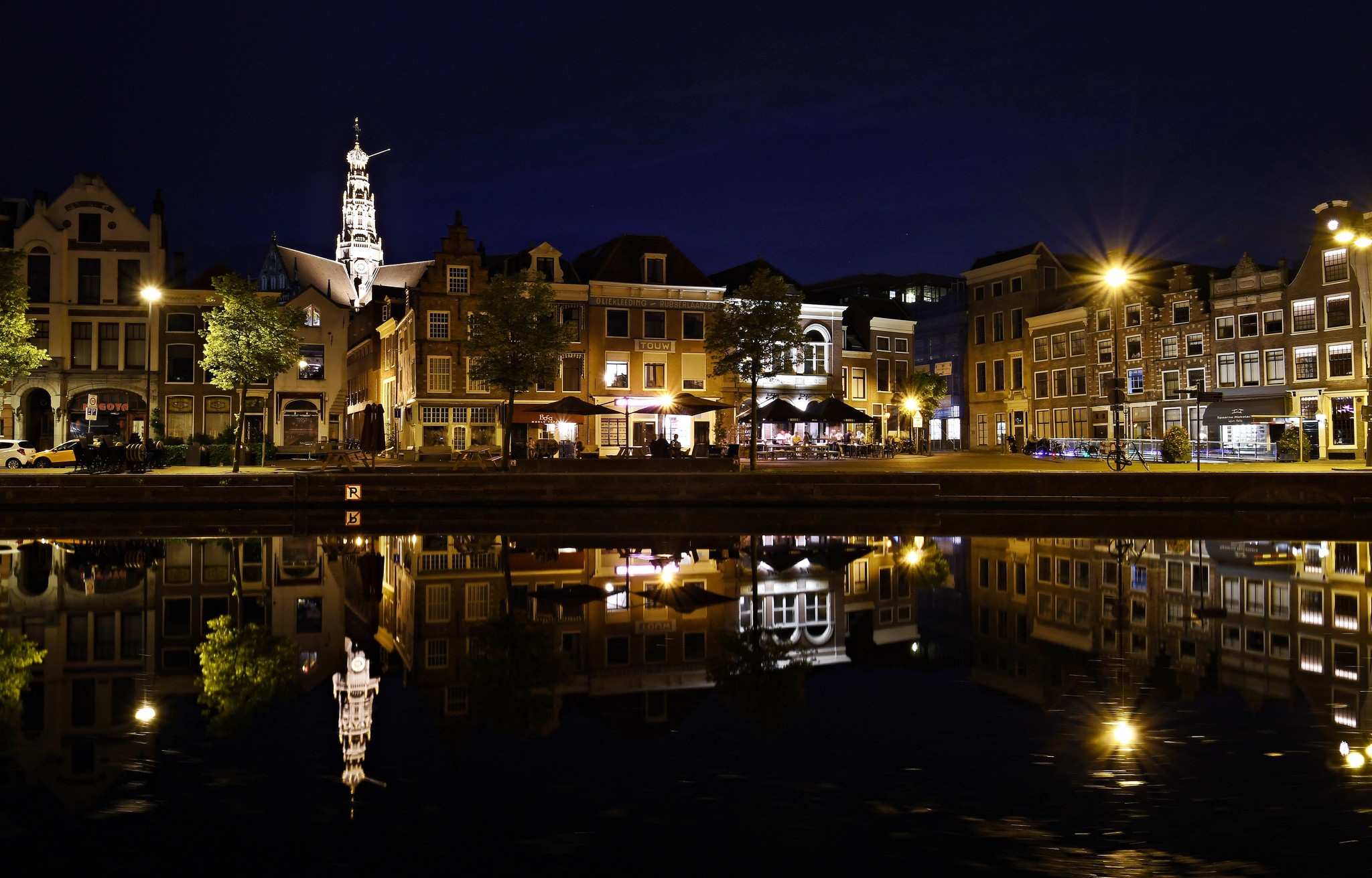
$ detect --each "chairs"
[62,539,168,581]
[682,443,740,458]
[73,432,167,473]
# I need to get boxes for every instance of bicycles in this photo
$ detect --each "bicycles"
[1036,436,1069,460]
[1107,436,1150,471]
[1073,434,1099,458]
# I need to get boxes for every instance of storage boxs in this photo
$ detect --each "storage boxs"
[385,446,398,459]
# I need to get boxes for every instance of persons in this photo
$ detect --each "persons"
[650,433,659,458]
[670,434,691,455]
[656,433,675,459]
[574,437,585,459]
[774,428,867,455]
[526,437,537,459]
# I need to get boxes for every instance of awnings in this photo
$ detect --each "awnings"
[1202,398,1290,425]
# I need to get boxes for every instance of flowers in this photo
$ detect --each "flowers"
[1279,426,1313,457]
[1160,425,1190,459]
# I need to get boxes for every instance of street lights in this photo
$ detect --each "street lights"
[1333,227,1372,466]
[1109,539,1138,747]
[135,565,157,721]
[1103,266,1128,471]
[1122,396,1131,440]
[905,397,918,441]
[623,395,673,459]
[140,285,161,448]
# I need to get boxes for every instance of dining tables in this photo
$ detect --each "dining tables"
[668,446,684,458]
[532,439,559,459]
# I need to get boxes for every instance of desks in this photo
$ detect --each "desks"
[618,446,646,459]
[317,535,361,558]
[452,534,497,555]
[320,450,370,473]
[451,447,498,472]
[755,438,896,460]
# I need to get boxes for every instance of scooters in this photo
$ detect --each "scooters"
[1023,433,1050,455]
[1007,434,1018,453]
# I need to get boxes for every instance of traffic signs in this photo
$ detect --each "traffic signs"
[1200,392,1224,403]
[1172,389,1197,393]
[1186,385,1197,390]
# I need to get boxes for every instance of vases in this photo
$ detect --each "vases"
[1278,452,1311,463]
[1161,453,1192,463]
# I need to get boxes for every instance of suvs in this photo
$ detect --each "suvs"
[28,438,99,468]
[0,439,36,469]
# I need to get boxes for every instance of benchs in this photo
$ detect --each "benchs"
[418,446,453,461]
[485,455,502,462]
[275,444,313,460]
[576,445,599,458]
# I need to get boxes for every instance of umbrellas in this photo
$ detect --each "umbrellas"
[629,393,739,417]
[523,395,621,416]
[804,395,877,424]
[737,397,816,423]
[526,538,880,624]
